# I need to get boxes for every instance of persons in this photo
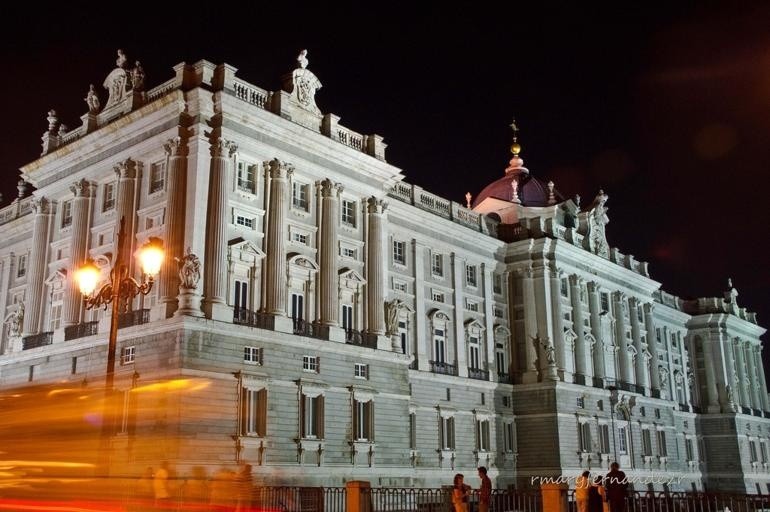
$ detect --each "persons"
[172,246,201,288]
[129,61,146,91]
[451,473,472,511]
[587,474,609,512]
[84,84,98,112]
[573,470,592,512]
[604,461,629,511]
[137,460,255,511]
[116,49,128,69]
[297,49,310,69]
[476,464,493,511]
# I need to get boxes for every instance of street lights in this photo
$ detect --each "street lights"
[74,236,166,478]
